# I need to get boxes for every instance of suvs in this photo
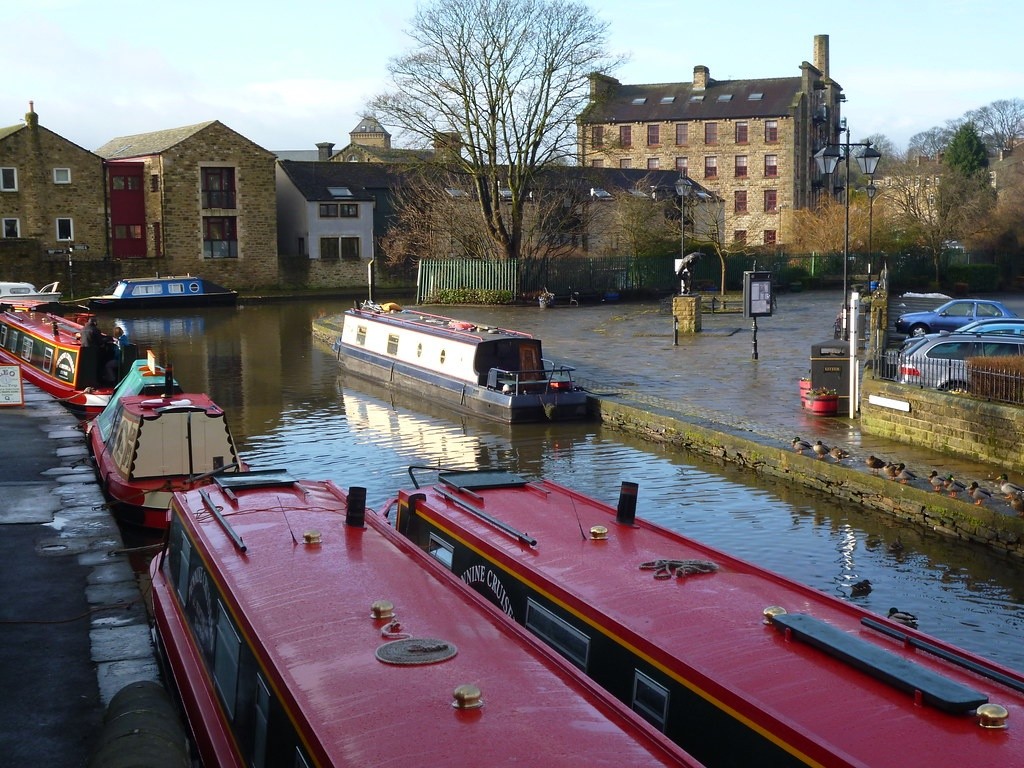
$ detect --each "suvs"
[898,330,1024,394]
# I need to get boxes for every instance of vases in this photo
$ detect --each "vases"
[540,301,546,308]
[799,380,811,405]
[812,397,838,416]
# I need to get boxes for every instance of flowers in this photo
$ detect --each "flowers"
[540,294,551,304]
[809,387,837,395]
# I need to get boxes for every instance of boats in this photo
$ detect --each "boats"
[0,301,139,407]
[148,465,708,768]
[0,281,63,304]
[330,299,587,425]
[376,464,1024,768]
[88,349,253,531]
[88,272,239,308]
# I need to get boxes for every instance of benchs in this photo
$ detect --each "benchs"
[700,295,715,313]
[569,288,601,306]
[715,295,744,310]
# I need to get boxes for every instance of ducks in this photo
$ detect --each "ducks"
[791,437,851,463]
[886,607,920,627]
[850,579,873,596]
[884,461,917,484]
[866,455,887,475]
[928,471,991,506]
[889,536,904,551]
[996,473,1024,517]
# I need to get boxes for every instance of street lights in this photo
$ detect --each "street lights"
[864,176,876,296]
[674,168,693,258]
[813,124,881,342]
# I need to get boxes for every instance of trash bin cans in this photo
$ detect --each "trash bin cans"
[811,337,853,418]
[839,302,866,349]
[743,270,775,315]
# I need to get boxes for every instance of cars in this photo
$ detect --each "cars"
[894,299,1018,339]
[902,316,1024,350]
